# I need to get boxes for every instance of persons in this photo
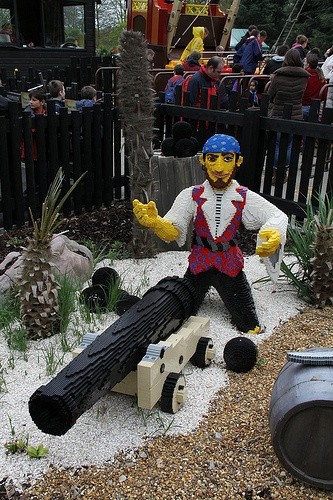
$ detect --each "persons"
[132,133,289,335]
[0,25,333,196]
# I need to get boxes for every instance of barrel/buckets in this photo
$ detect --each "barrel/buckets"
[268,346,333,490]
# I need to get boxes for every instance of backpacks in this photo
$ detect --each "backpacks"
[165,78,185,104]
